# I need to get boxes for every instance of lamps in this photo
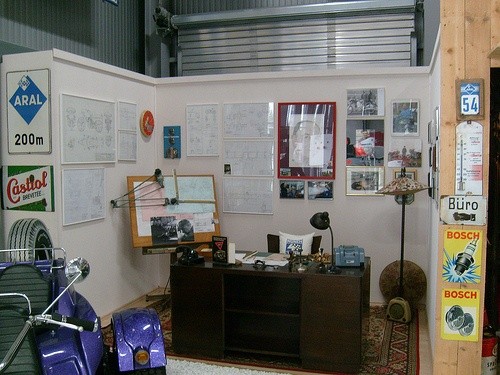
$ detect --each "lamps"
[375,168,432,323]
[311,212,341,274]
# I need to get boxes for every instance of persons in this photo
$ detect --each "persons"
[402,146,406,156]
[360,130,369,142]
[346,137,356,154]
[367,90,376,104]
[177,219,194,242]
[361,90,368,103]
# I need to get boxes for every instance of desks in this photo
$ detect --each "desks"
[169,251,371,375]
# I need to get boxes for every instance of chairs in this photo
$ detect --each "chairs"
[267,234,323,255]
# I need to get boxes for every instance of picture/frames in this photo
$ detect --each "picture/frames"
[428,108,438,201]
[277,102,336,180]
[388,139,423,168]
[345,120,384,166]
[309,181,333,200]
[345,169,385,196]
[392,101,420,136]
[393,169,417,181]
[279,180,305,199]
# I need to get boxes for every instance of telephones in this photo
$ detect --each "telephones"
[175,246,205,265]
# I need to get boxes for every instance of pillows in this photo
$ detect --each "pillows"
[279,231,315,256]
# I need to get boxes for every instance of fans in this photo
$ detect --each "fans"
[380,260,427,318]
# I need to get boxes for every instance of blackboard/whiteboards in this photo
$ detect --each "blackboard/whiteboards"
[61,168,107,227]
[118,101,137,132]
[222,178,274,214]
[59,92,118,164]
[118,132,138,161]
[222,102,274,139]
[222,140,275,177]
[185,104,220,157]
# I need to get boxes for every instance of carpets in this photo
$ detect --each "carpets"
[100,297,420,375]
